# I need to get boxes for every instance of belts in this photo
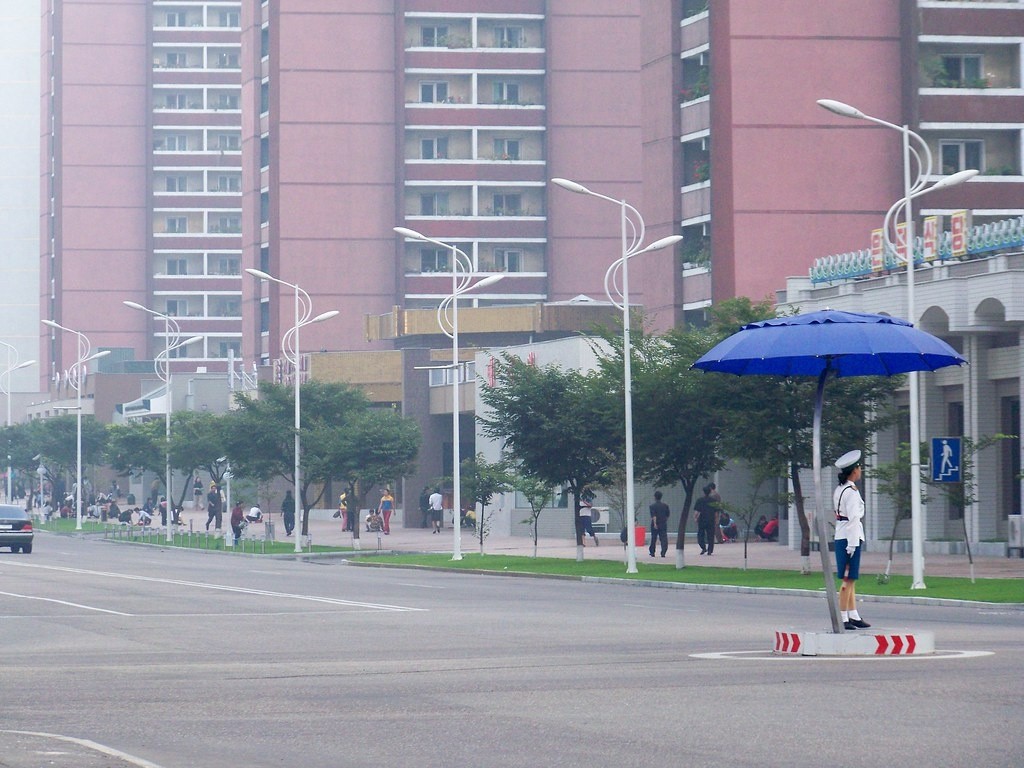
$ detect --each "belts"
[836,516,863,522]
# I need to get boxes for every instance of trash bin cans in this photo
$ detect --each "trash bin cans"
[127,494,136,505]
[101,508,107,522]
[264,520,275,542]
[1008,514,1024,559]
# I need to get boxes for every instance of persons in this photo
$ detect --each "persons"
[159,492,188,526]
[833,450,871,630]
[280,490,295,536]
[193,477,205,511]
[452,505,476,528]
[694,487,719,555]
[205,485,223,532]
[720,511,737,542]
[338,486,359,532]
[428,487,443,534]
[754,515,769,543]
[649,490,670,557]
[231,500,264,539]
[579,491,599,548]
[763,512,779,541]
[704,482,723,544]
[620,518,640,545]
[418,487,432,528]
[365,489,396,535]
[11,476,160,526]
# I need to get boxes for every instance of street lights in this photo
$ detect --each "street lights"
[816,97,980,589]
[123,301,204,542]
[393,226,505,560]
[0,342,36,504]
[551,177,683,573]
[42,320,112,530]
[244,268,340,553]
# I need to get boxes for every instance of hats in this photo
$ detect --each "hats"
[834,450,863,469]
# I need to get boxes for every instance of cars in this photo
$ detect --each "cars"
[0,505,34,553]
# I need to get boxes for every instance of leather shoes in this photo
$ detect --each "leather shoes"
[843,621,856,629]
[849,617,870,627]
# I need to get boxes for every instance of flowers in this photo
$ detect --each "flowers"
[690,159,706,181]
[675,66,707,103]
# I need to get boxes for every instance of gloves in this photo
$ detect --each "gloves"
[846,546,855,558]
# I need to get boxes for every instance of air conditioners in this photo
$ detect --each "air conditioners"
[1008,514,1024,547]
[590,507,609,523]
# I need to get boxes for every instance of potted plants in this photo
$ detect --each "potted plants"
[683,241,696,270]
[1000,164,1014,176]
[422,259,507,273]
[431,206,535,217]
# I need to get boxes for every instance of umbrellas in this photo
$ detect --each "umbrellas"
[688,306,970,656]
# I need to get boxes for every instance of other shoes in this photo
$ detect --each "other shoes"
[433,531,436,534]
[649,553,655,557]
[661,554,665,557]
[438,530,440,533]
[701,548,707,554]
[707,553,711,555]
[594,537,599,545]
